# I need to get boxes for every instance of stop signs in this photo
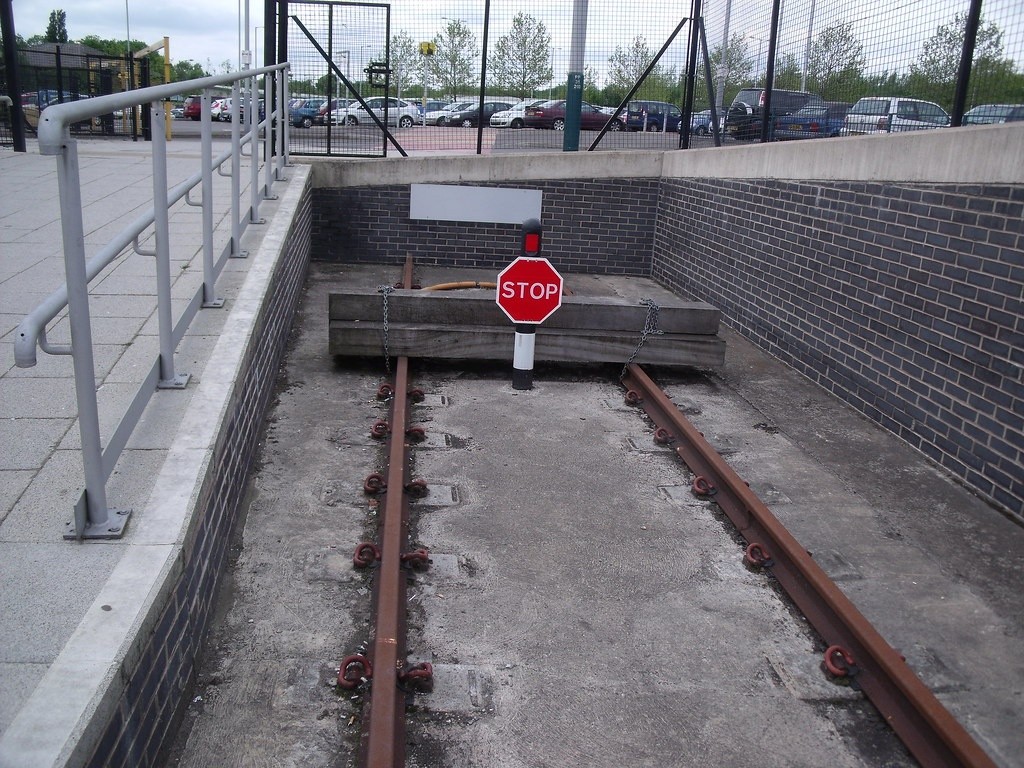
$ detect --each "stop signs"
[495,256,564,325]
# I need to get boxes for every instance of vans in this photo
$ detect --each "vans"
[626,100,682,132]
[414,101,450,113]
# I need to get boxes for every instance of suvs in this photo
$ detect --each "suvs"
[725,88,823,141]
[220,97,252,122]
[288,98,326,128]
[184,94,215,121]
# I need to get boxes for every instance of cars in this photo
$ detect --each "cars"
[239,99,265,123]
[962,104,1024,126]
[314,99,358,125]
[677,113,714,136]
[839,96,952,136]
[211,99,225,121]
[774,102,855,139]
[490,99,548,128]
[112,108,130,119]
[444,102,514,128]
[523,100,625,132]
[323,96,421,128]
[417,102,474,127]
[20,89,103,126]
[169,107,185,120]
[707,115,726,133]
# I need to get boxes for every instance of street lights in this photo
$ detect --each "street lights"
[749,36,762,87]
[359,45,372,96]
[548,47,562,99]
[254,26,264,79]
[442,16,467,101]
